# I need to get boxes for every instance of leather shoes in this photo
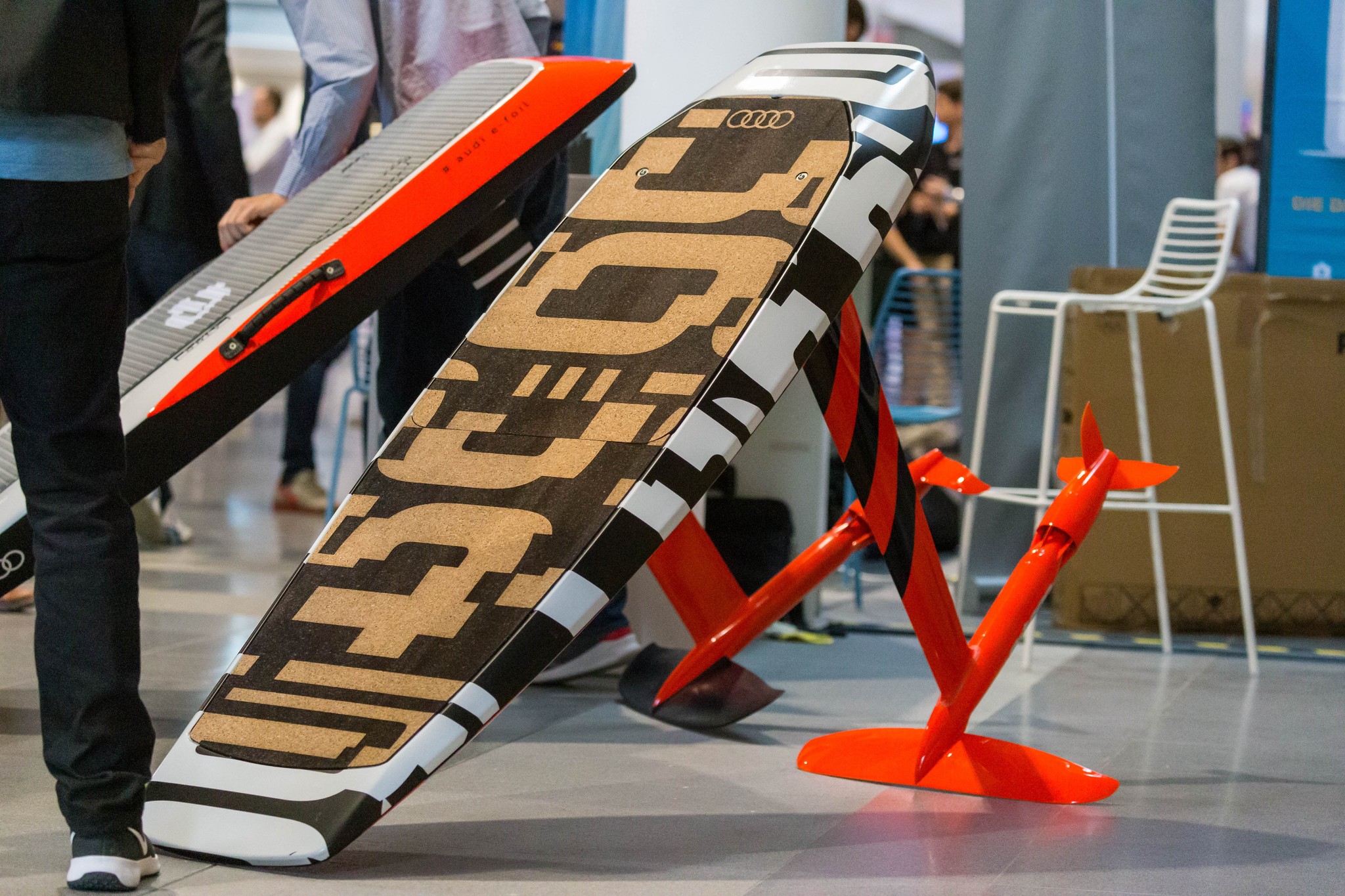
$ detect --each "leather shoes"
[0,587,35,612]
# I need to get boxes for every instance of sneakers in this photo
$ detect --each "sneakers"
[132,480,194,546]
[276,469,341,515]
[527,618,642,683]
[65,827,161,892]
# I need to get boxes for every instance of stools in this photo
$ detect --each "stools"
[957,196,1260,674]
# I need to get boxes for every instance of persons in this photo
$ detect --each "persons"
[844,1,1270,416]
[272,62,373,512]
[124,0,253,548]
[1,0,168,893]
[218,2,571,439]
[242,85,281,168]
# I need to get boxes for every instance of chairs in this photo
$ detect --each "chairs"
[842,269,963,610]
[324,319,375,525]
